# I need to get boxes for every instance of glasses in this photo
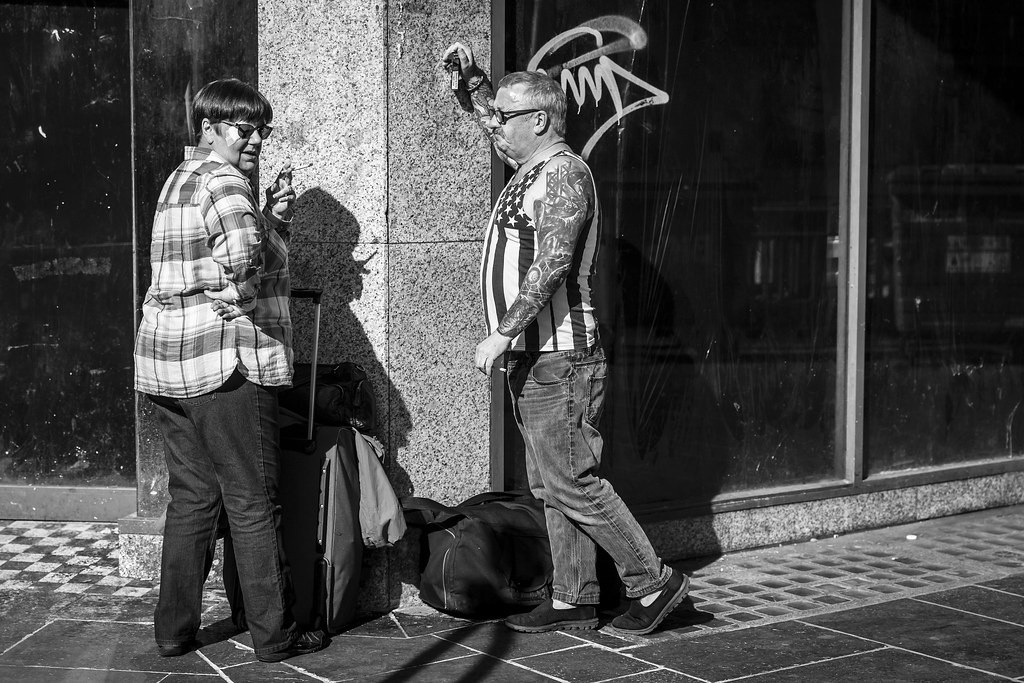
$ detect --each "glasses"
[211,120,273,138]
[488,109,544,125]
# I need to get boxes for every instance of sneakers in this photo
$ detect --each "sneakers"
[255,630,329,662]
[158,634,195,657]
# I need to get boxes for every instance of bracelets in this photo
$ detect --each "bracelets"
[464,71,484,92]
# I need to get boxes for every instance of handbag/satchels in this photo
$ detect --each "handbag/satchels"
[421,491,553,613]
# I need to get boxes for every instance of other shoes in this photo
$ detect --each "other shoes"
[611,568,690,633]
[505,596,600,632]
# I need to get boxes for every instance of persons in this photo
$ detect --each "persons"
[442,41,690,636]
[133,78,327,663]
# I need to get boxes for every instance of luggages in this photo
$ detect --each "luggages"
[224,287,367,636]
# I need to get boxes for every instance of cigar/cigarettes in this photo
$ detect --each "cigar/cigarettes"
[492,365,507,372]
[281,162,313,175]
